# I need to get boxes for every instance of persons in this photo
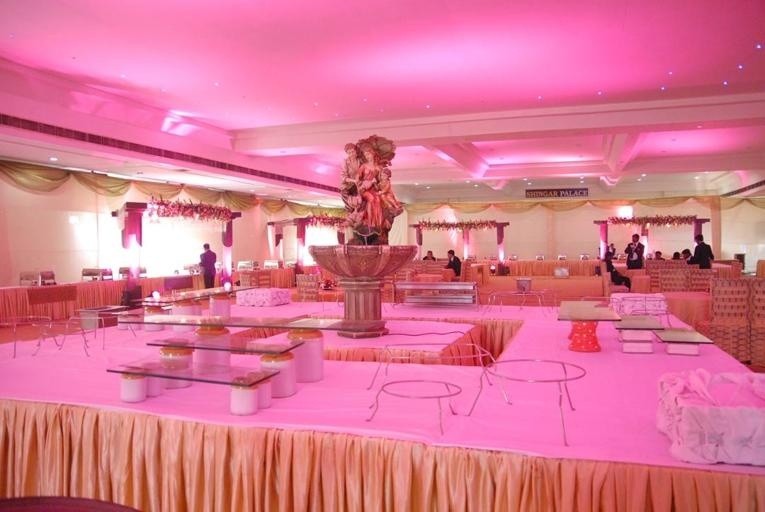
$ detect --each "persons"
[423,251,435,261]
[127,273,137,292]
[605,243,615,259]
[341,143,399,232]
[625,234,644,269]
[200,243,216,288]
[445,250,461,276]
[673,234,714,269]
[654,251,665,261]
[607,260,630,288]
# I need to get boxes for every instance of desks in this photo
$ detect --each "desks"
[0,254,764,510]
